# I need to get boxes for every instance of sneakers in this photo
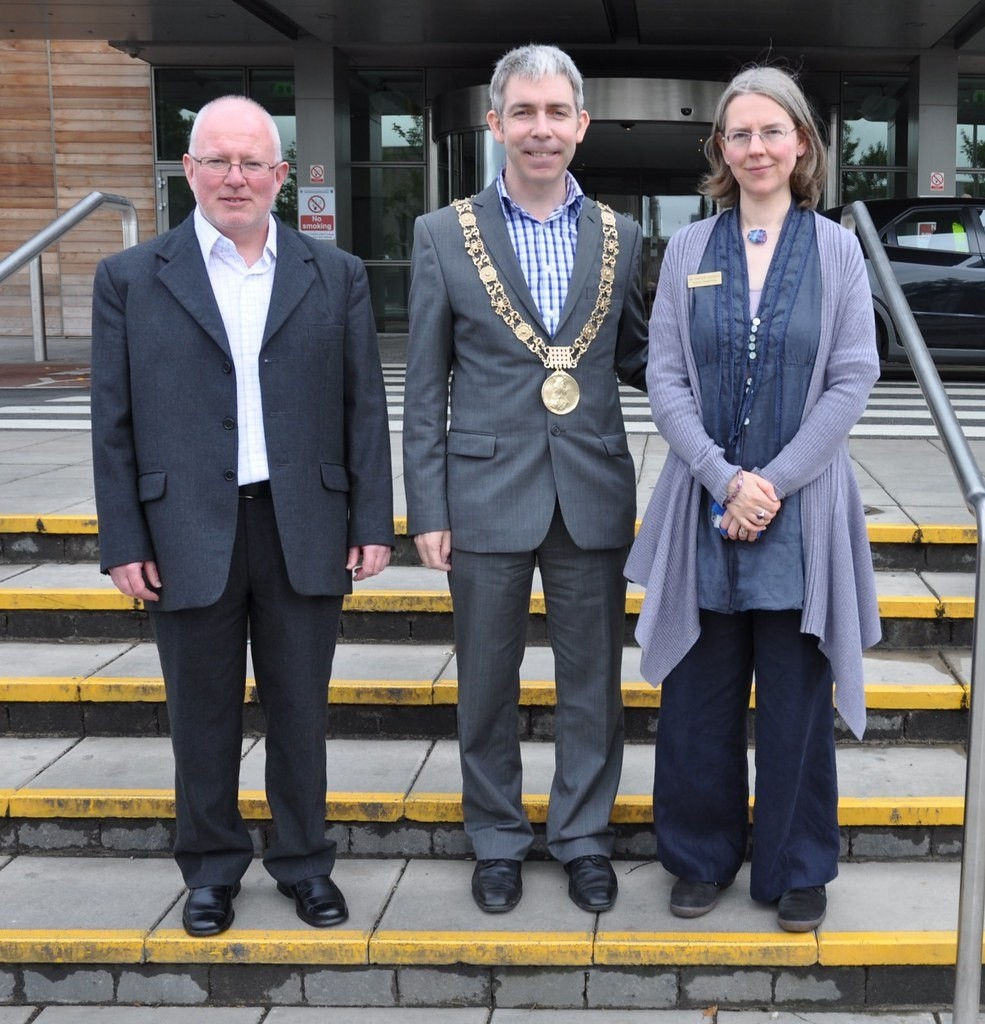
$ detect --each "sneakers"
[670,876,736,917]
[777,885,829,932]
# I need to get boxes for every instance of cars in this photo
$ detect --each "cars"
[817,197,985,369]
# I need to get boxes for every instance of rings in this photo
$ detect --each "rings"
[739,527,748,535]
[757,509,765,519]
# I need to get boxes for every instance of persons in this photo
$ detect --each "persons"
[90,93,396,937]
[403,43,649,916]
[647,61,880,927]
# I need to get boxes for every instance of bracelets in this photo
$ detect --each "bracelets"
[723,469,743,505]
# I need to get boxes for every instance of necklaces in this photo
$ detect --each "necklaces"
[747,229,768,244]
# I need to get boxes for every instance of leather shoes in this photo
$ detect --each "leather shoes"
[182,880,242,936]
[566,855,618,913]
[278,876,349,926]
[469,858,522,912]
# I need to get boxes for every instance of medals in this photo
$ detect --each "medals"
[452,193,617,415]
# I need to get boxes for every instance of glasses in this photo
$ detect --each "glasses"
[722,125,798,146]
[187,153,284,179]
